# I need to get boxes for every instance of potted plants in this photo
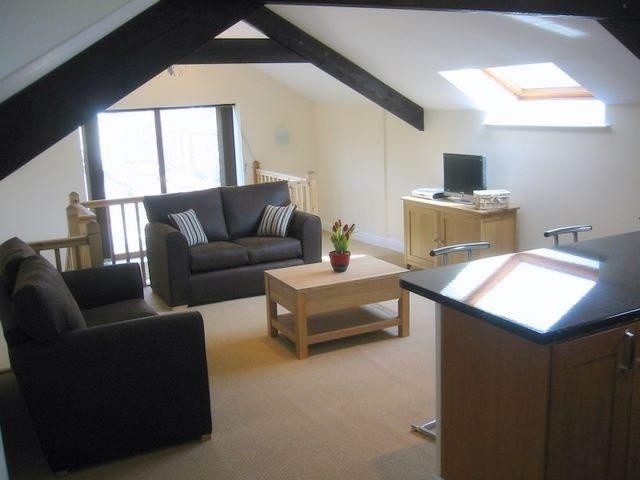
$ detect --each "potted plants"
[328,220,355,272]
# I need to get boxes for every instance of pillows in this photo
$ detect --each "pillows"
[256,203,297,237]
[168,209,208,247]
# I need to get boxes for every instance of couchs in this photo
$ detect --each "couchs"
[144,181,322,307]
[0,237,212,477]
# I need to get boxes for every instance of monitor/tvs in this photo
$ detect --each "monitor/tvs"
[443,152,484,205]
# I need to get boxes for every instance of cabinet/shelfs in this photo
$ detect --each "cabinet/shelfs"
[440,303,640,480]
[400,196,520,271]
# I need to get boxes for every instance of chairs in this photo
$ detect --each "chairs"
[412,240,490,438]
[543,225,592,247]
